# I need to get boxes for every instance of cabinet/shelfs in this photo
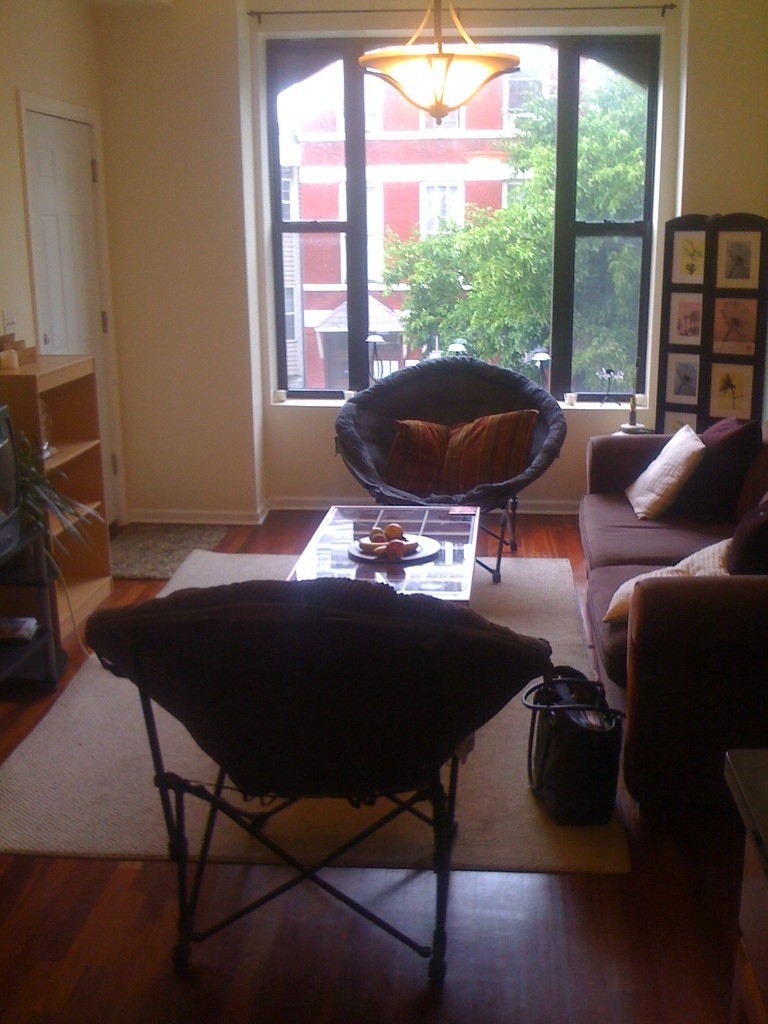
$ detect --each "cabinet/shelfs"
[0,352,115,642]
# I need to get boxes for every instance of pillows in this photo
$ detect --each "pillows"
[624,422,705,521]
[382,418,452,503]
[600,536,732,625]
[442,409,539,497]
[726,492,767,576]
[685,418,762,524]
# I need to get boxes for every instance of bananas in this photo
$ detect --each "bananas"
[355,537,418,555]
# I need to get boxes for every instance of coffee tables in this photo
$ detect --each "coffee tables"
[285,507,483,611]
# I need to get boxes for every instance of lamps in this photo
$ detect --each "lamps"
[357,0,521,127]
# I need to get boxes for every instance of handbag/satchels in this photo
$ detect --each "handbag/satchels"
[515,661,622,830]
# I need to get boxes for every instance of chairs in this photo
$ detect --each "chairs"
[334,356,566,585]
[85,577,551,986]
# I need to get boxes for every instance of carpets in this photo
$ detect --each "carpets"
[1,551,633,876]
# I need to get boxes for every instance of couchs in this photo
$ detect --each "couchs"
[578,413,768,835]
